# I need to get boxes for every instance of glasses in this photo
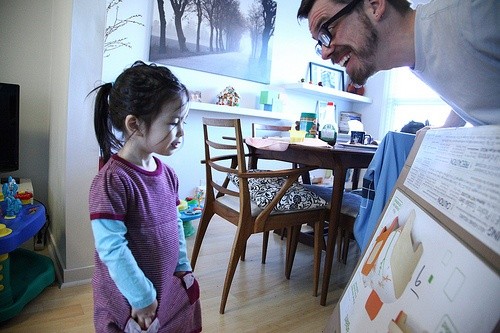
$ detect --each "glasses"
[314,0,364,57]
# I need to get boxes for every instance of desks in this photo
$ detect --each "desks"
[241,136,382,306]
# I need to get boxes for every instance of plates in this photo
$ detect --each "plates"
[339,142,380,148]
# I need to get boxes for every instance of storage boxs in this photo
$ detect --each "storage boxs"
[327,124,500,333]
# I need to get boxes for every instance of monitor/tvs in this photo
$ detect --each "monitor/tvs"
[0,83,20,172]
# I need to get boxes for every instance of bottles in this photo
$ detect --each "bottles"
[339,111,361,134]
[318,102,338,146]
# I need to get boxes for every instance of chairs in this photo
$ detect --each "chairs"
[190,116,328,314]
[251,121,312,263]
[286,131,421,291]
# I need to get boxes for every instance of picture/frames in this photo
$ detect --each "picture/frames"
[318,100,337,133]
[310,61,344,91]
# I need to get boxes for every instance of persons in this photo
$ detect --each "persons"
[297,0,500,139]
[88,61,203,333]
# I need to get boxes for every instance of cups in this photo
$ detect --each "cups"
[291,121,300,131]
[351,130,372,145]
[289,130,307,144]
